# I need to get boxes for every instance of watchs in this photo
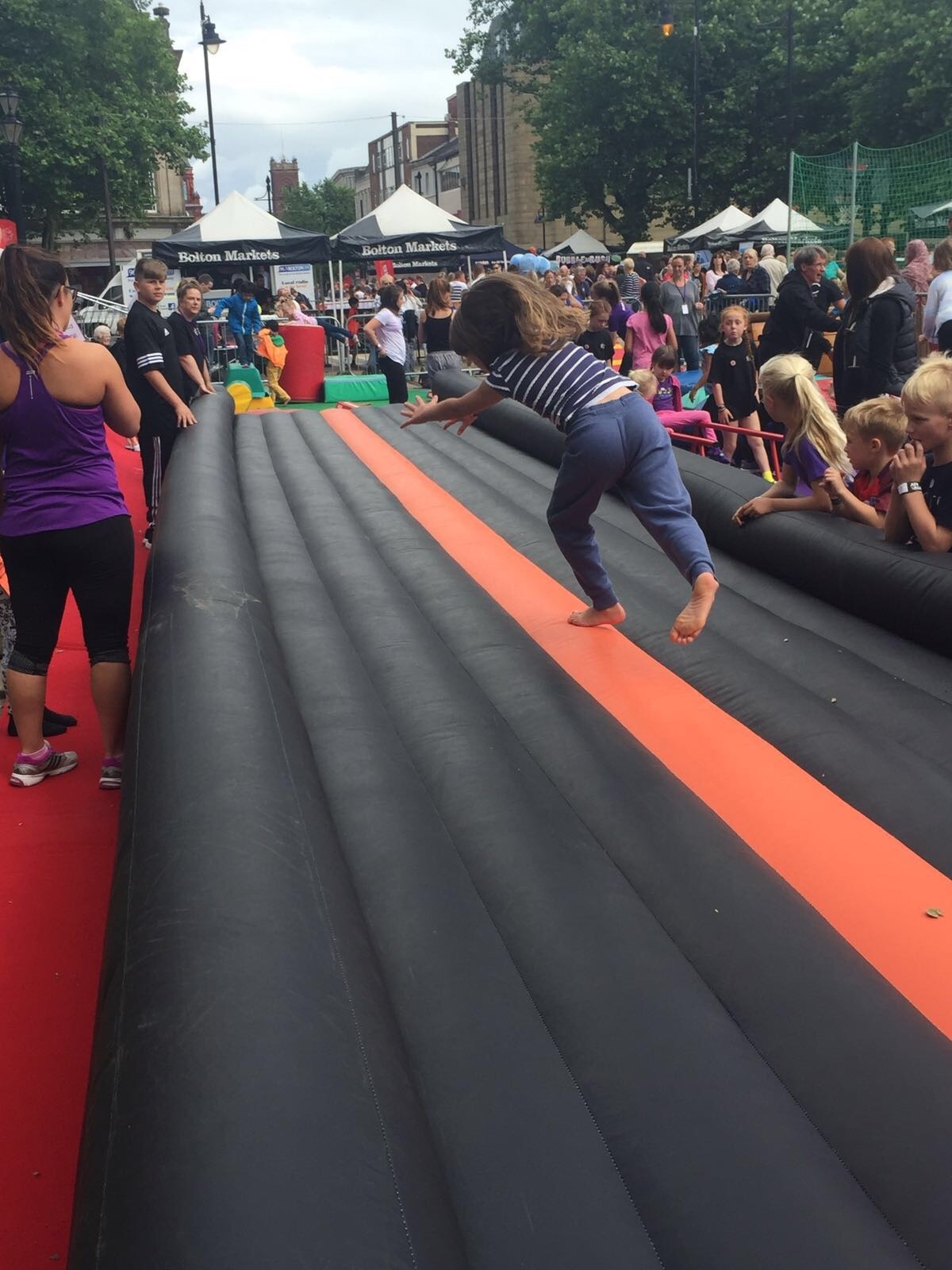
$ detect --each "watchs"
[375,343,380,348]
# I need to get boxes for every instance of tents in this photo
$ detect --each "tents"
[153,191,342,373]
[367,198,851,276]
[334,183,508,372]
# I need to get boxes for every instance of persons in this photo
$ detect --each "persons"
[0,237,952,789]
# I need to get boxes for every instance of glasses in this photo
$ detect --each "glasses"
[55,284,78,303]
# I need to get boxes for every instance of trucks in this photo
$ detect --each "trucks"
[75,248,321,350]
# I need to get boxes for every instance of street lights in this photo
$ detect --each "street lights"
[0,80,26,246]
[660,0,700,231]
[194,0,226,206]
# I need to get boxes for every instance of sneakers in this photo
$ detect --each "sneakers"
[99,758,124,789]
[11,738,78,788]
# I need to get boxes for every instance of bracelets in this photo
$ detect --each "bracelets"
[897,481,924,496]
[717,403,726,412]
[376,346,383,354]
